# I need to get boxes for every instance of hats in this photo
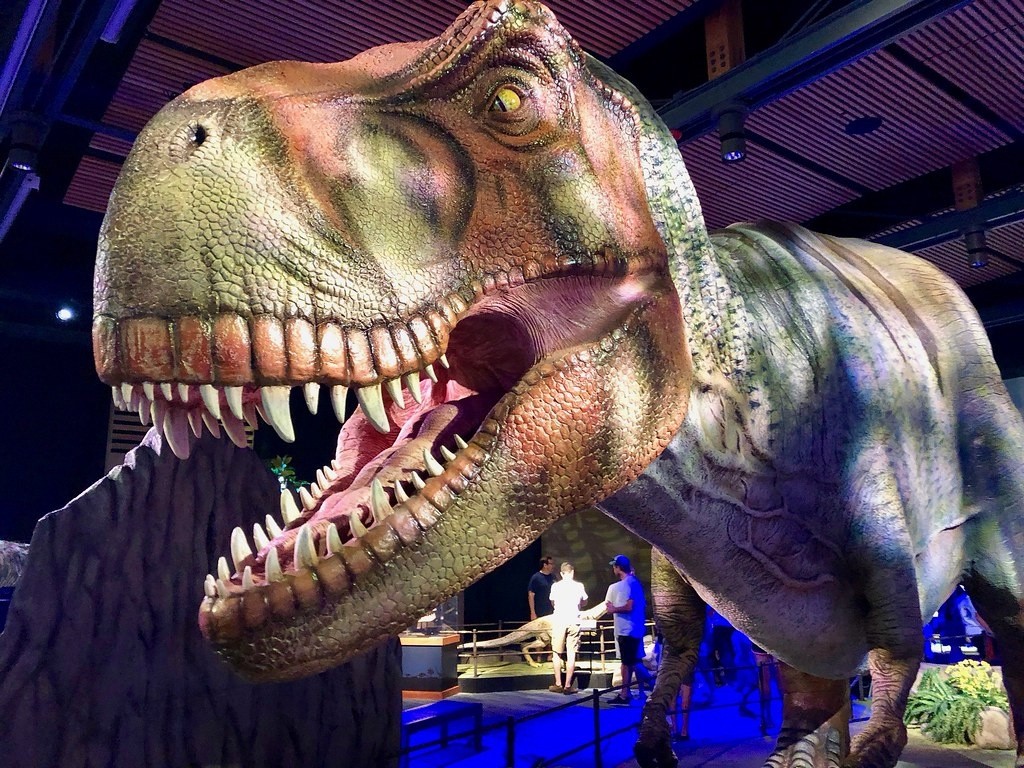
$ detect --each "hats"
[609,554,630,568]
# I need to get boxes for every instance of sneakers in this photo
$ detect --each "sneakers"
[606,694,629,707]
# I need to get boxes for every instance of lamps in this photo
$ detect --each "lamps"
[718,110,747,164]
[965,232,989,268]
[8,122,41,173]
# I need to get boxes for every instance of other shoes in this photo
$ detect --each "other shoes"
[549,685,563,692]
[564,687,578,694]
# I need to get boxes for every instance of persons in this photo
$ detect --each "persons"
[605,555,655,706]
[669,627,757,743]
[922,587,998,664]
[527,557,553,662]
[549,562,589,694]
